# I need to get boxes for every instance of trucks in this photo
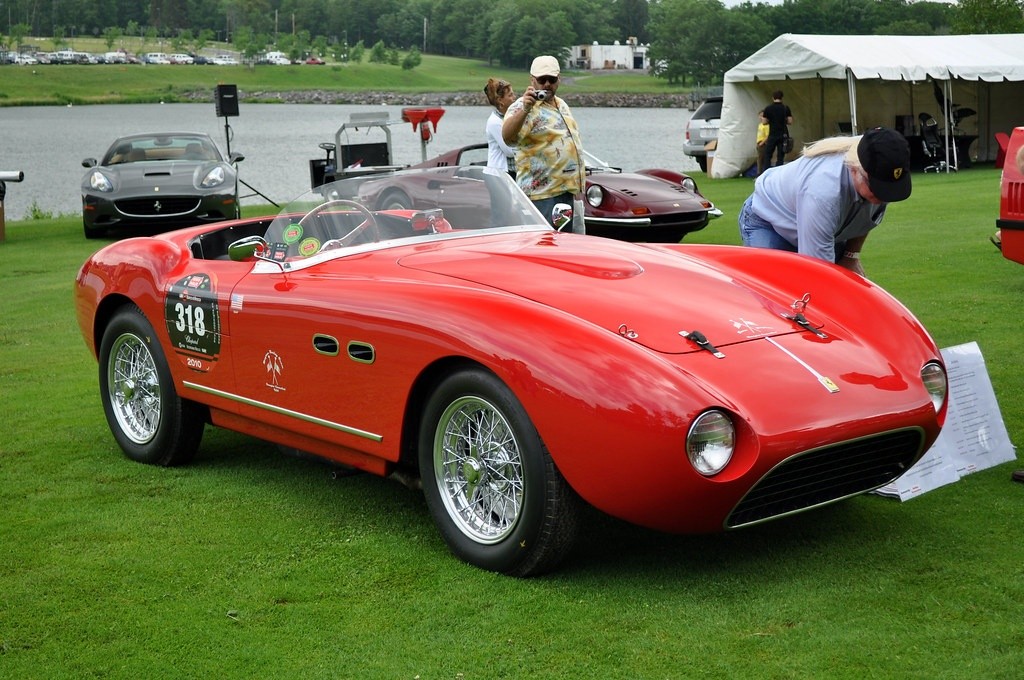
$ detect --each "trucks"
[266,52,291,66]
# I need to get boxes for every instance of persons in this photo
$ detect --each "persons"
[756,91,794,176]
[481,76,519,185]
[502,54,587,236]
[740,127,911,266]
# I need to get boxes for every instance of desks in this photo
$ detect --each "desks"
[906,134,979,172]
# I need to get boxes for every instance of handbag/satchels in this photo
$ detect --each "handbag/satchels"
[783,137,793,153]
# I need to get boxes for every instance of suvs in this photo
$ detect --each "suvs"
[683,95,724,173]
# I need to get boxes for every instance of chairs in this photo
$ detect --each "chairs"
[128,149,146,162]
[183,143,205,159]
[916,112,958,174]
[838,121,853,134]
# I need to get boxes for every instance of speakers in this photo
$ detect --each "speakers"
[214,84,239,117]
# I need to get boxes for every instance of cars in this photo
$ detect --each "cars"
[81,130,245,239]
[75,166,950,581]
[0,50,239,65]
[359,142,726,244]
[989,127,1024,265]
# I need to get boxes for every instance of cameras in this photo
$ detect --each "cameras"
[532,90,548,100]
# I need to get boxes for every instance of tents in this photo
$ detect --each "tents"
[710,30,1024,181]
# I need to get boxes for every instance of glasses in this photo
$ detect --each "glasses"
[533,75,559,84]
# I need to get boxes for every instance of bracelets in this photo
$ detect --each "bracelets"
[521,106,529,114]
[843,250,860,259]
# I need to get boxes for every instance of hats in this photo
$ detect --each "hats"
[858,129,912,202]
[531,55,560,76]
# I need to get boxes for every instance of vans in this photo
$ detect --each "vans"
[306,57,326,64]
